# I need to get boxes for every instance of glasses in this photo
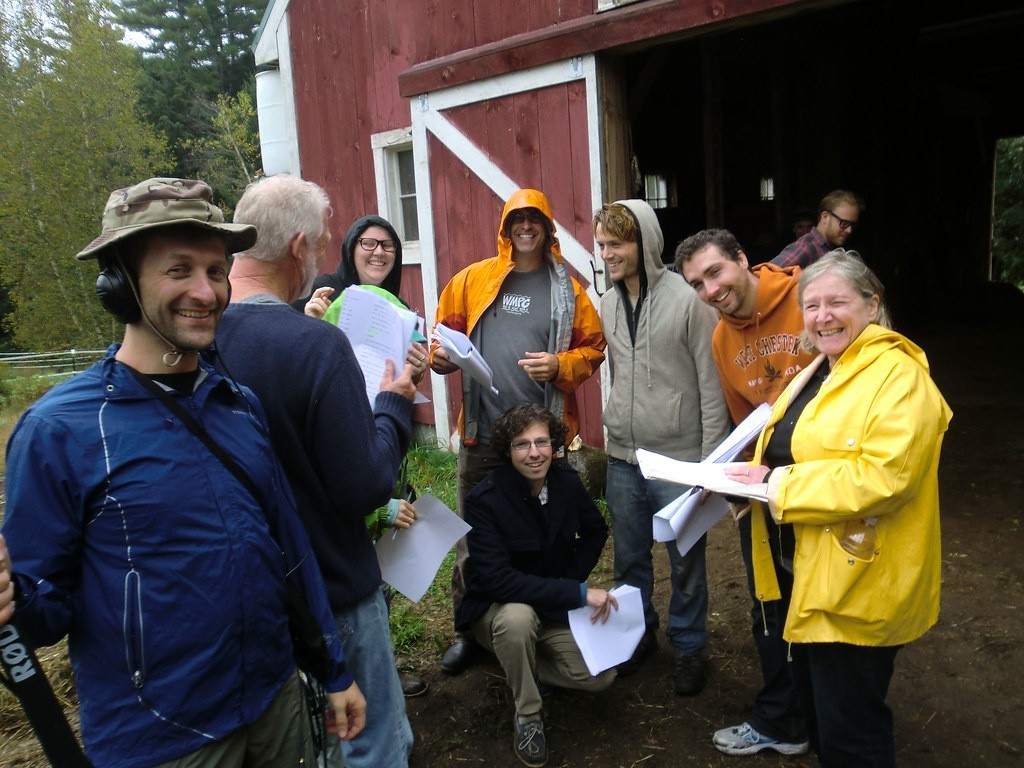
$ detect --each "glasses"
[822,210,856,229]
[512,212,544,223]
[358,238,397,252]
[508,437,553,450]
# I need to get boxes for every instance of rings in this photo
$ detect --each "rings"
[420,355,426,361]
[418,362,423,368]
[745,469,750,476]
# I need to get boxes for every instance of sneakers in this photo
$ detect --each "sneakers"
[616,631,658,669]
[712,722,809,755]
[513,711,549,767]
[673,649,706,695]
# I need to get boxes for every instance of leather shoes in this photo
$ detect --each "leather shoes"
[398,672,429,697]
[440,642,480,672]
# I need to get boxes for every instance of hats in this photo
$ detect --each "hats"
[76,178,258,260]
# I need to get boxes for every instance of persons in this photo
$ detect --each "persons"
[0,174,430,768]
[431,189,954,767]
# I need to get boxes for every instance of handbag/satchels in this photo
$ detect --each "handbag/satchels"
[297,667,327,750]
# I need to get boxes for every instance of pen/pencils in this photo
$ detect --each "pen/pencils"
[392,489,415,541]
[690,486,701,496]
